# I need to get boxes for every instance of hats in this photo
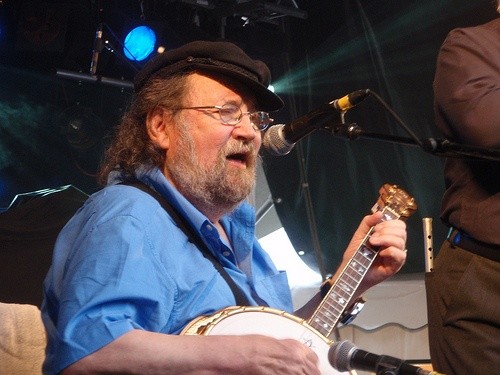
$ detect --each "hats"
[133,41,284,111]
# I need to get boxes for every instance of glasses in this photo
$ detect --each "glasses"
[173,102,275,130]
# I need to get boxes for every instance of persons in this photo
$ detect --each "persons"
[39,39,408,375]
[425,0,500,375]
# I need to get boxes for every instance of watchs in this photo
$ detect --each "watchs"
[320,273,366,327]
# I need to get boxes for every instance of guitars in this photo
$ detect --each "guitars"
[177,183,418,375]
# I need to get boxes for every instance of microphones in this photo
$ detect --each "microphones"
[328,340,442,375]
[263,89,371,156]
[90,22,104,75]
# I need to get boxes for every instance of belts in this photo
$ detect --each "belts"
[447,224,500,263]
